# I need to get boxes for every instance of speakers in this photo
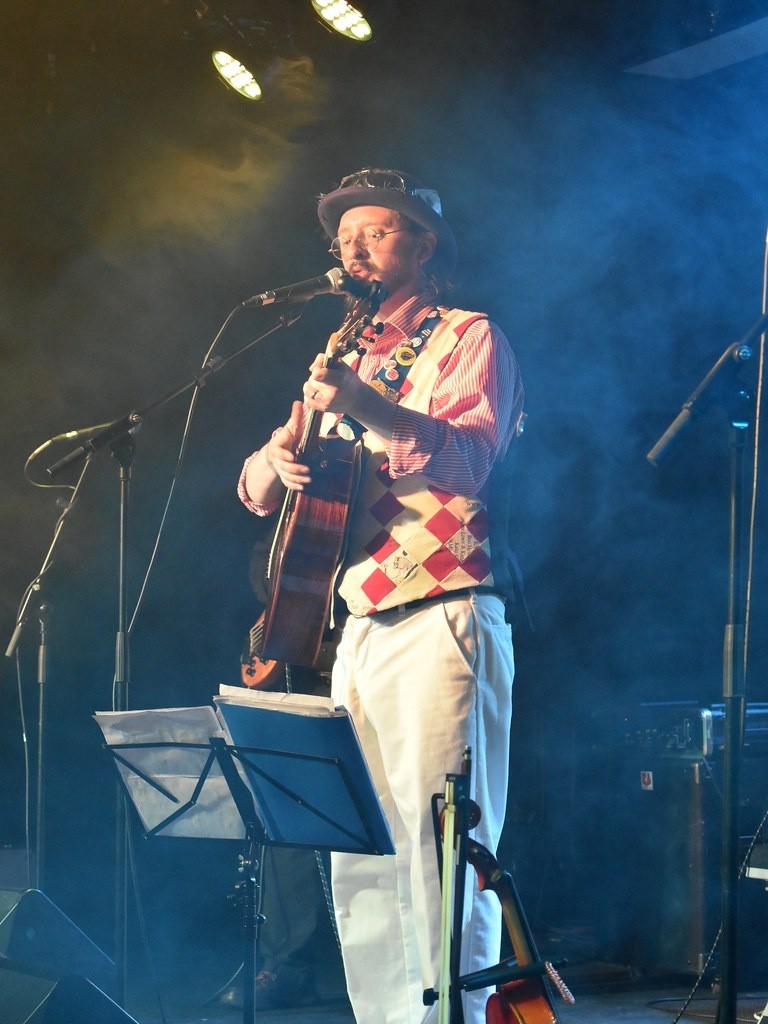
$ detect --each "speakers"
[611,750,768,988]
[0,843,138,1024]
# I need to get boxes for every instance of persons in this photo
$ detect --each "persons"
[221,167,525,1024]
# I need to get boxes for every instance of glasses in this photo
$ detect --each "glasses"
[329,229,417,260]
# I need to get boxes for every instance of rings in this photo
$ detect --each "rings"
[311,390,320,400]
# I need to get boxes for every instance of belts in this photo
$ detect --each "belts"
[378,585,502,612]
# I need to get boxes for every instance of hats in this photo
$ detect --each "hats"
[318,168,458,263]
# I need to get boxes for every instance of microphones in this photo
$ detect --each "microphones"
[242,267,349,307]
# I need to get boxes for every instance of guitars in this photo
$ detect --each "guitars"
[238,408,530,689]
[258,277,387,668]
